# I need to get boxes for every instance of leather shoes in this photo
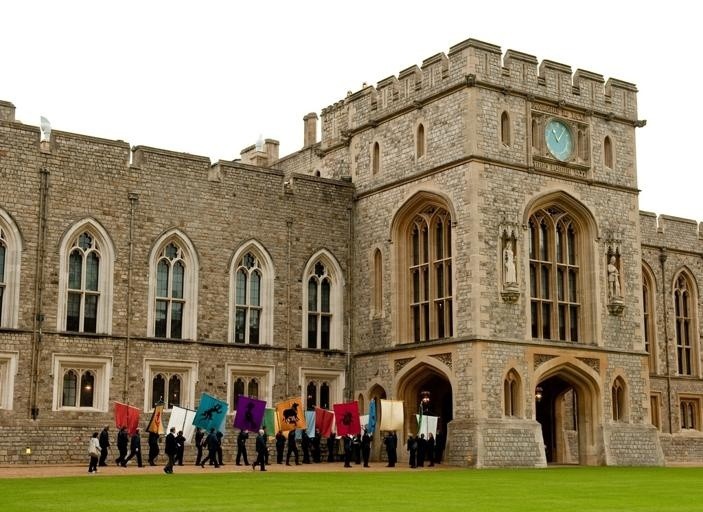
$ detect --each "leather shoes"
[138,459,184,467]
[364,465,371,468]
[99,463,108,467]
[344,464,352,468]
[252,463,256,471]
[115,459,128,467]
[196,460,251,468]
[385,462,396,467]
[265,459,336,467]
[164,468,173,473]
[260,469,267,471]
[409,463,435,468]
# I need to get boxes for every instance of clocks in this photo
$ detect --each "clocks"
[539,116,578,164]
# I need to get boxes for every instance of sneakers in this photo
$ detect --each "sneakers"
[88,470,99,474]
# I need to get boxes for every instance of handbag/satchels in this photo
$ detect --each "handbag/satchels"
[88,445,101,459]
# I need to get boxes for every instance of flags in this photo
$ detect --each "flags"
[192,393,227,432]
[333,400,362,435]
[367,399,377,432]
[415,404,423,434]
[233,395,335,440]
[165,406,197,443]
[379,399,404,431]
[146,406,165,435]
[416,414,438,442]
[114,401,140,433]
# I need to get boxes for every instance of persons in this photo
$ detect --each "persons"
[502,240,517,283]
[195,427,226,468]
[609,256,621,299]
[88,425,186,474]
[236,425,270,472]
[276,429,445,468]
